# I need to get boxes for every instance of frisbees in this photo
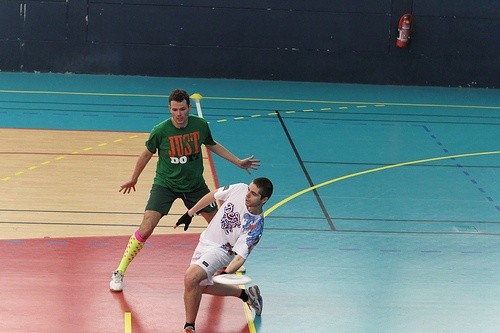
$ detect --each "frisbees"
[214,275,252,285]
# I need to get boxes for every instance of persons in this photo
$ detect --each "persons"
[173,176,274,333]
[110,88,261,293]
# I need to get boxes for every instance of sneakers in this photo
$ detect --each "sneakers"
[110,270,123,291]
[237,266,246,272]
[245,284,263,316]
[183,326,195,333]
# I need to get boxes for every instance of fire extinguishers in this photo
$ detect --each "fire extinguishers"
[396,13,413,48]
